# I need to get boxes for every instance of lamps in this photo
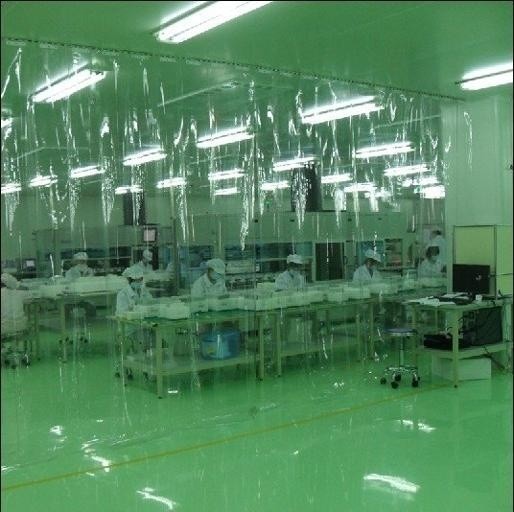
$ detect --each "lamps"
[1,2,514,209]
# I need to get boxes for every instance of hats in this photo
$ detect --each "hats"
[142,249,154,262]
[286,254,304,266]
[365,248,381,264]
[206,258,226,276]
[0,272,18,289]
[425,225,441,252]
[122,265,144,279]
[73,251,89,261]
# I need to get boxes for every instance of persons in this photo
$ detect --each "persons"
[134,249,155,270]
[66,251,93,281]
[1,273,31,335]
[117,264,158,324]
[426,226,446,258]
[353,249,383,285]
[191,259,227,296]
[418,245,447,278]
[274,253,309,290]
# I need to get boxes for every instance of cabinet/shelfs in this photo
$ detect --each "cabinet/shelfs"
[408,289,513,387]
[0,209,449,400]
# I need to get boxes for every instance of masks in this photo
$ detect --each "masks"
[131,282,144,291]
[210,273,222,282]
[289,270,299,277]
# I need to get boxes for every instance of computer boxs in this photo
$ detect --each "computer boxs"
[462,306,503,346]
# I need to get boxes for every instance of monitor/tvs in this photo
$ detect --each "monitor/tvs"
[452,264,490,303]
[141,227,158,243]
[23,258,36,271]
